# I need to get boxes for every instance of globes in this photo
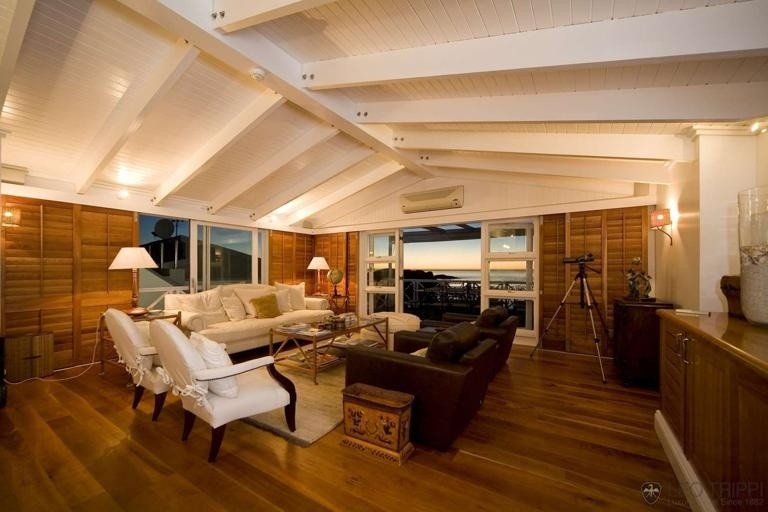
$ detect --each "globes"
[327,268,343,296]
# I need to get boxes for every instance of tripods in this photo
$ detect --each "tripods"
[530,273,614,384]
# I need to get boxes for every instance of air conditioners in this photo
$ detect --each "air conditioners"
[399,185,463,214]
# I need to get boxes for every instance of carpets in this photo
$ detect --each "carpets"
[240,331,395,448]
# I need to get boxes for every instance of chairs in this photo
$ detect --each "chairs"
[104,307,173,422]
[149,317,297,462]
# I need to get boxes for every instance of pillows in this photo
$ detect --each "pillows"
[248,293,282,320]
[188,328,242,402]
[220,291,247,323]
[275,280,306,312]
[274,288,295,314]
[236,287,277,315]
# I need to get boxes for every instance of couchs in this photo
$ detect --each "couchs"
[360,310,421,351]
[416,306,519,376]
[344,321,497,450]
[162,284,335,355]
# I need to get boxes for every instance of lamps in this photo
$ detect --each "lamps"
[106,246,159,318]
[306,255,331,297]
[648,207,674,246]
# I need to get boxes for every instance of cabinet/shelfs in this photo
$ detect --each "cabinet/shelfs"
[654,308,768,512]
[612,296,673,391]
